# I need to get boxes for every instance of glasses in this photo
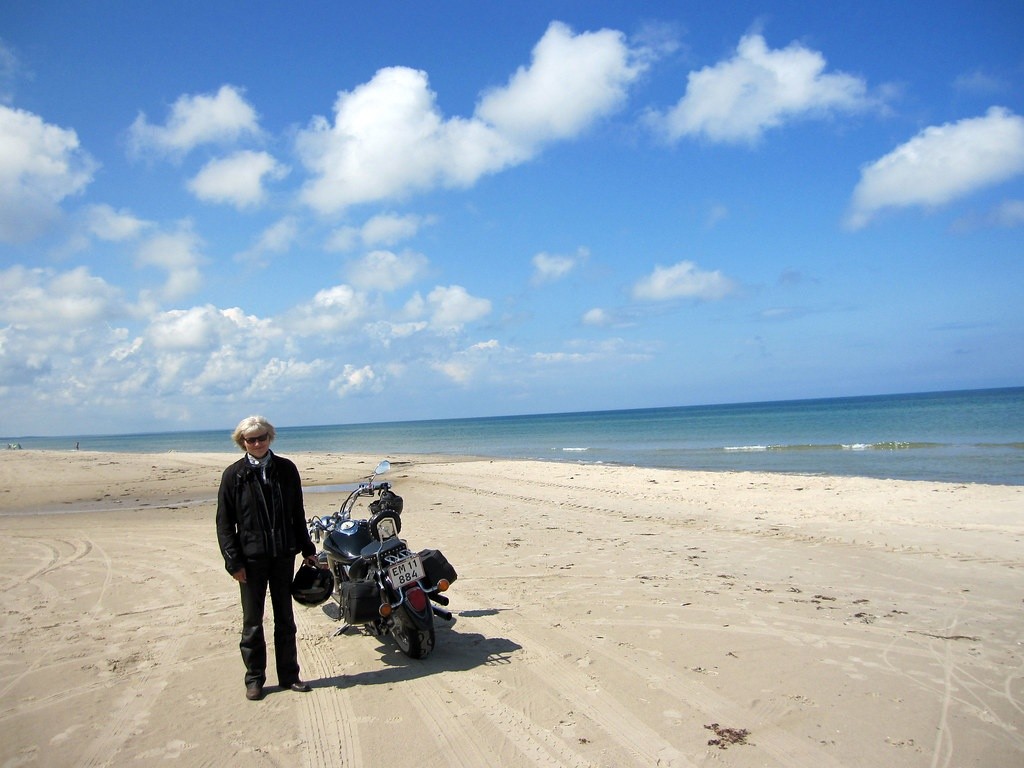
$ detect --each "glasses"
[241,430,271,445]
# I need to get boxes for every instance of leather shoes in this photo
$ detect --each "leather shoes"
[244,684,262,699]
[279,679,310,691]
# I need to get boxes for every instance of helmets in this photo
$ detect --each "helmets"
[368,491,404,516]
[291,561,335,606]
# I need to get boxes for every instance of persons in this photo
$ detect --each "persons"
[215,416,317,700]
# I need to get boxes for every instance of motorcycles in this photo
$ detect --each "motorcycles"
[303,459,457,659]
